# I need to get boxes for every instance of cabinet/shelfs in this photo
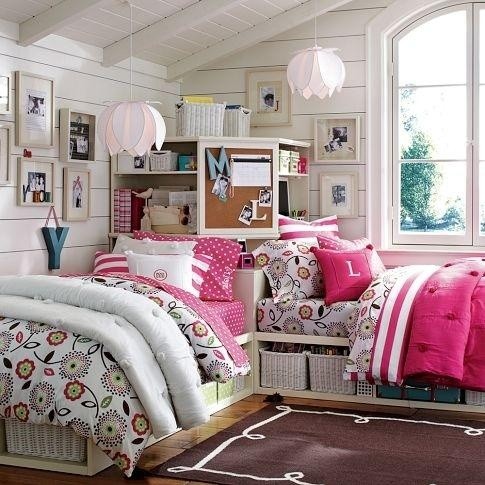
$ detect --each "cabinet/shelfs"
[107,136,311,253]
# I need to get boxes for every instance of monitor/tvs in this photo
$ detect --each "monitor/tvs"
[279,177,290,216]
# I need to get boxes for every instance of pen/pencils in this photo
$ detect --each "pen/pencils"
[292,209,308,217]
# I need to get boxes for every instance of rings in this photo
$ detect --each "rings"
[265,93,273,106]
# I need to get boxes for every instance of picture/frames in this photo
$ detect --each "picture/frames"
[318,170,360,219]
[0,126,11,186]
[15,70,57,148]
[0,75,11,115]
[17,157,56,207]
[197,136,279,241]
[62,166,92,222]
[245,67,291,127]
[313,115,360,164]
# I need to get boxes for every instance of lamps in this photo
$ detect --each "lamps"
[286,0,346,100]
[97,0,166,157]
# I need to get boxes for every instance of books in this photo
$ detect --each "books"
[147,184,197,207]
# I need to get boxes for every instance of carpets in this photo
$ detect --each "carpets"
[121,391,484,485]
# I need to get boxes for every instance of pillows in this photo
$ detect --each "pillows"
[276,214,340,242]
[93,250,213,298]
[316,232,387,281]
[133,229,243,302]
[124,250,193,294]
[253,237,324,305]
[113,232,197,253]
[310,243,374,307]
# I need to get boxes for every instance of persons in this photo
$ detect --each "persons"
[216,177,229,196]
[241,191,271,222]
[29,174,44,191]
[30,97,43,113]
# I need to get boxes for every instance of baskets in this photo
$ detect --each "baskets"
[175,101,251,137]
[291,217,306,221]
[465,389,485,405]
[259,344,357,395]
[4,415,86,463]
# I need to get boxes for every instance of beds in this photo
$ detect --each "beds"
[256,267,485,414]
[0,298,255,478]
